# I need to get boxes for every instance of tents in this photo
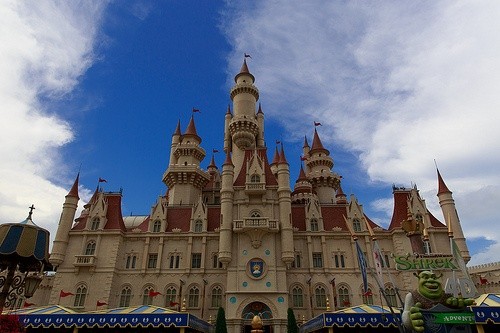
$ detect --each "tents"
[0,304,216,333]
[302,293,500,333]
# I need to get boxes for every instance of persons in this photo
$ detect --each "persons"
[401,270,475,333]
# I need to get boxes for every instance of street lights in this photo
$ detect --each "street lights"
[0,204,49,333]
